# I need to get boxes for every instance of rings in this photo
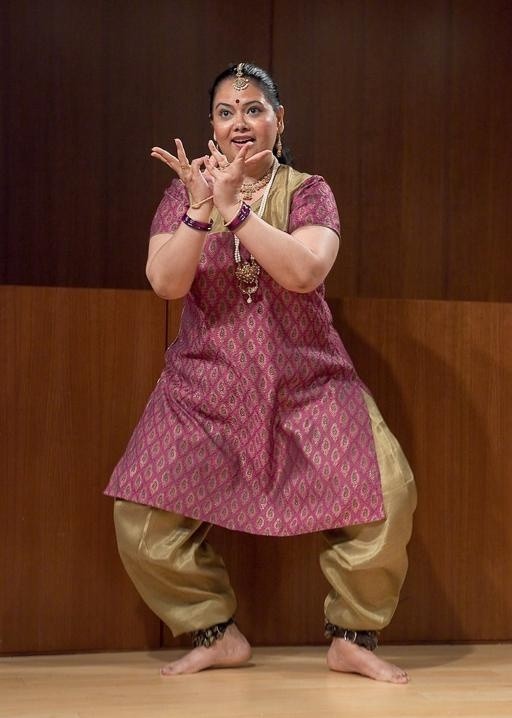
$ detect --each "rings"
[224,162,230,168]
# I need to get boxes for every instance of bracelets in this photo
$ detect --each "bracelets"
[221,200,251,230]
[180,210,215,232]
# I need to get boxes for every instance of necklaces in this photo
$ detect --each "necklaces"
[223,160,284,305]
[237,159,277,201]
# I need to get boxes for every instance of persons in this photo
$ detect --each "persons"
[102,60,422,686]
